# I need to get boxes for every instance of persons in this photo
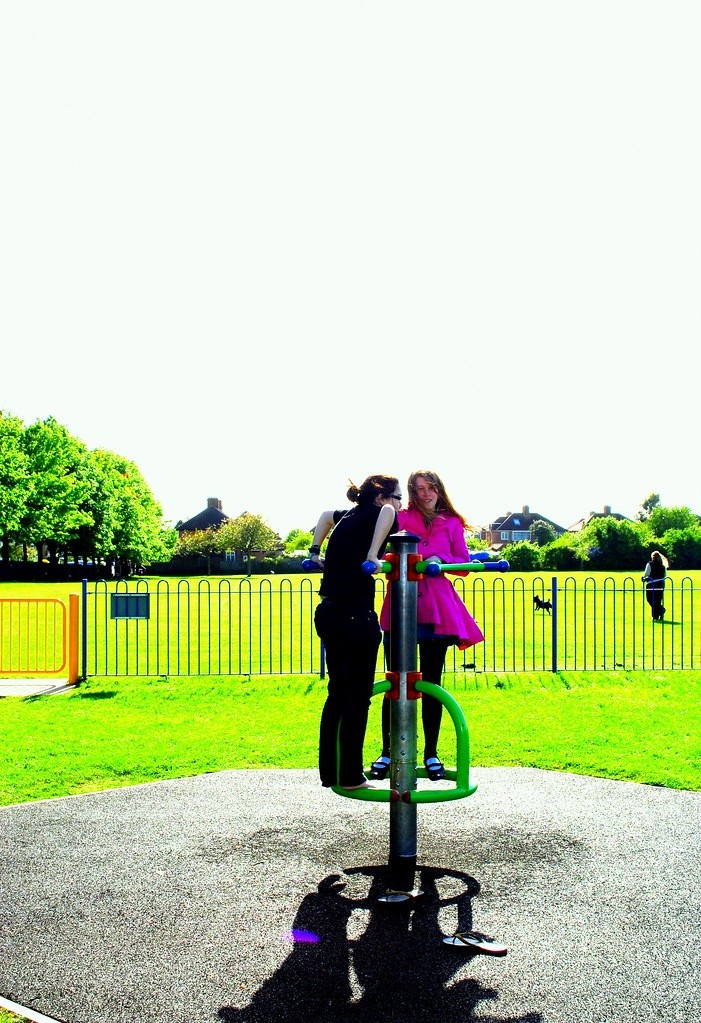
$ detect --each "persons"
[309,474,402,790]
[642,551,669,620]
[369,470,484,781]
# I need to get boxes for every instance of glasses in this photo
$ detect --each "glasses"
[416,485,435,493]
[386,493,401,502]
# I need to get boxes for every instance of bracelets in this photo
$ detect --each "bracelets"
[309,545,321,559]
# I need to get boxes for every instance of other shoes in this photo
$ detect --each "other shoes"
[660,608,666,620]
[424,757,444,781]
[654,616,658,621]
[371,755,391,779]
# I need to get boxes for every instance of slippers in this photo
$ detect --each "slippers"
[376,888,424,905]
[442,932,508,956]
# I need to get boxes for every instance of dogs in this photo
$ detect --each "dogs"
[533,595,553,615]
